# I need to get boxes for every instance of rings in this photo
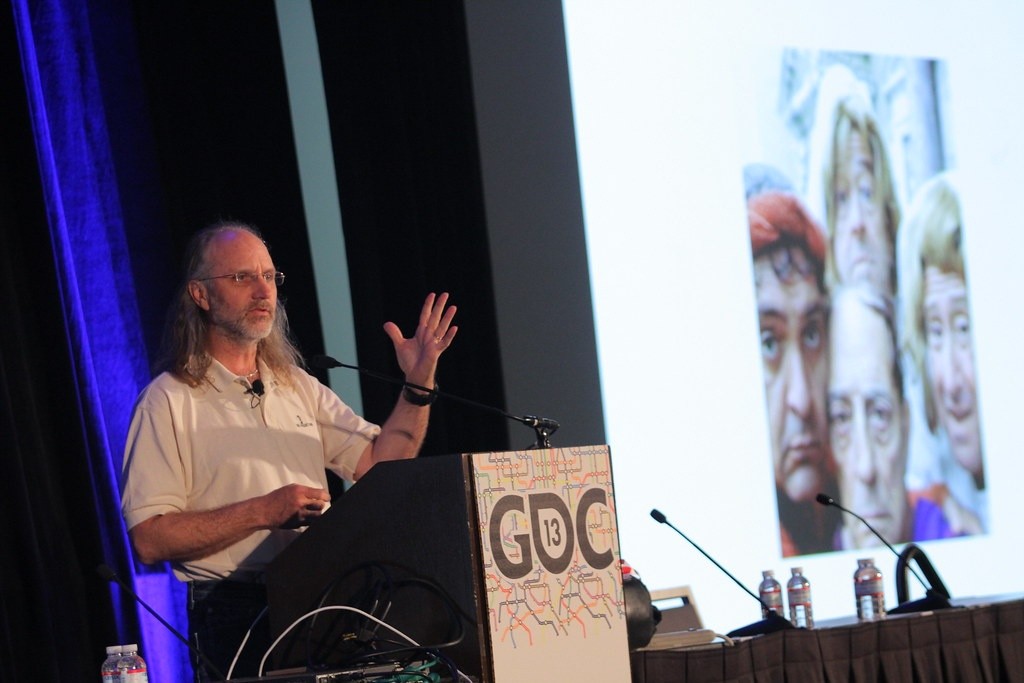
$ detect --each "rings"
[434,333,442,340]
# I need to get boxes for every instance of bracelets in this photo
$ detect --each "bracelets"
[401,383,440,405]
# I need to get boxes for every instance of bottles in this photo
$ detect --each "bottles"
[759,570,784,619]
[786,567,814,629]
[853,558,886,624]
[117,644,149,683]
[101,645,122,683]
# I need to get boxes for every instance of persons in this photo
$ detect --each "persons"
[747,62,990,558]
[120,225,458,683]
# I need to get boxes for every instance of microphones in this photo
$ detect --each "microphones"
[650,509,798,637]
[313,356,560,428]
[96,565,227,681]
[251,379,265,395]
[814,493,951,615]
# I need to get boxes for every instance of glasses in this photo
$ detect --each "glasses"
[198,270,285,288]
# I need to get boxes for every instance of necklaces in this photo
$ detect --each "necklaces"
[244,369,259,378]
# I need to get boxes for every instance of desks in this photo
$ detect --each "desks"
[630,599,1024,683]
